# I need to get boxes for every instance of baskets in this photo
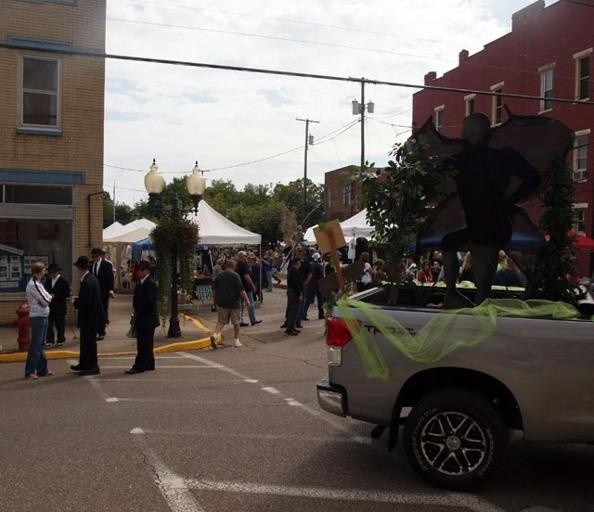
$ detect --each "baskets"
[195,284,213,301]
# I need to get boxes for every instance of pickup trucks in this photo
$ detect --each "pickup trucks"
[313,282,593,487]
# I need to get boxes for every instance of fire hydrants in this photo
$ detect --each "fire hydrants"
[11,302,32,350]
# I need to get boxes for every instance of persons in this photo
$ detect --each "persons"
[41,261,72,349]
[192,248,373,349]
[23,260,56,381]
[66,255,103,377]
[425,112,541,308]
[121,258,160,375]
[89,248,115,340]
[288,225,307,246]
[283,208,294,242]
[355,248,584,301]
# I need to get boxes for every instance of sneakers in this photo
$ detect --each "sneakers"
[97,336,104,340]
[251,320,263,326]
[71,363,100,376]
[240,322,250,327]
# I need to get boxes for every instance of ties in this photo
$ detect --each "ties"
[94,262,98,277]
[138,279,142,287]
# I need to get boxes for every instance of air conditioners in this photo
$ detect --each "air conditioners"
[572,170,584,181]
[578,208,586,222]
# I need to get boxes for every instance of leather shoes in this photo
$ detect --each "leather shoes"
[125,362,155,374]
[280,316,310,336]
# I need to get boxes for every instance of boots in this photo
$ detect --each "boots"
[233,338,243,347]
[210,333,219,348]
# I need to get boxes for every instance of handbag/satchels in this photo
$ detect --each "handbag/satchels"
[126,315,138,339]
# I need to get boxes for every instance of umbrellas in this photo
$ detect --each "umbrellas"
[543,226,594,253]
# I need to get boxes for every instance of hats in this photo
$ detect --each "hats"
[238,250,249,257]
[46,263,63,271]
[24,373,40,381]
[56,342,64,348]
[72,255,93,268]
[44,343,54,350]
[37,370,55,377]
[89,248,105,256]
[291,256,301,263]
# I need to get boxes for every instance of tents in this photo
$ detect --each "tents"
[103,198,261,302]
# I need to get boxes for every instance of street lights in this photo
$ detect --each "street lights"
[348,77,376,215]
[143,158,207,340]
